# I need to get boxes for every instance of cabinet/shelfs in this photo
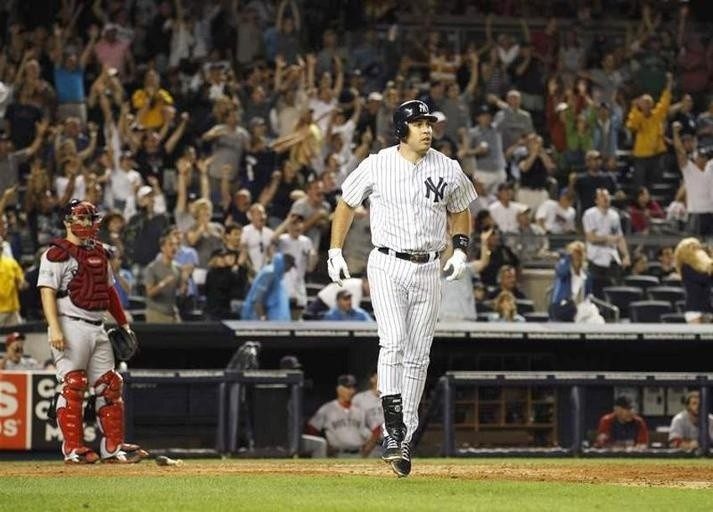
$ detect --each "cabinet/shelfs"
[414,379,560,454]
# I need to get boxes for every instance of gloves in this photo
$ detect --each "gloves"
[327,248,350,287]
[443,234,470,282]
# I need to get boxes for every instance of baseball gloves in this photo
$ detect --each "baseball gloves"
[110,328,139,359]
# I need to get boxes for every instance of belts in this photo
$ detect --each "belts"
[60,314,102,326]
[378,247,439,260]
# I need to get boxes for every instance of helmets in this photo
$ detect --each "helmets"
[58,200,101,239]
[393,100,437,137]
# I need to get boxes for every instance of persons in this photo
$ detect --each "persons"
[1,331,57,370]
[668,390,713,453]
[593,395,649,450]
[38,198,147,463]
[1,1,713,325]
[307,369,390,459]
[327,96,478,474]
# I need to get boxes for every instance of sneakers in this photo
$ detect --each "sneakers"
[65,451,100,463]
[101,451,140,463]
[383,437,403,461]
[391,443,411,478]
